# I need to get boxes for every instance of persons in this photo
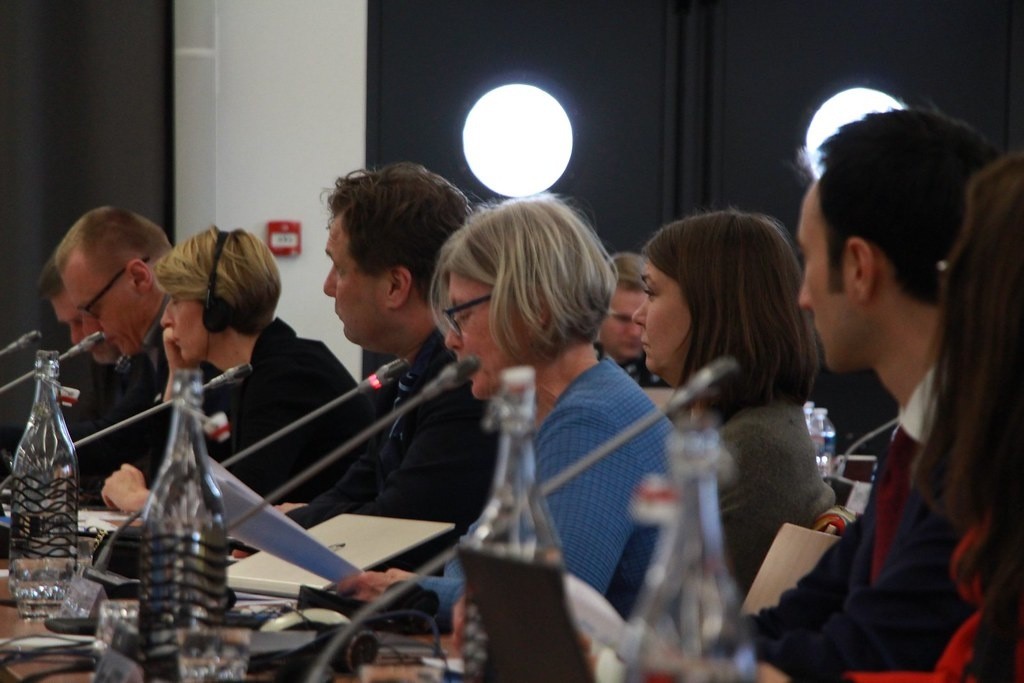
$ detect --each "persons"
[102,223,376,526]
[31,204,223,511]
[226,161,501,576]
[632,209,837,604]
[336,195,687,636]
[592,252,671,388]
[703,110,1024,683]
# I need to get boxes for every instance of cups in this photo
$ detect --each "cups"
[9,558,74,619]
[94,599,139,674]
[177,627,253,683]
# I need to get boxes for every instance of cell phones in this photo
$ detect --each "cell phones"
[44,616,100,636]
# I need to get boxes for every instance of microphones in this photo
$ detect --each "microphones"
[0,330,743,683]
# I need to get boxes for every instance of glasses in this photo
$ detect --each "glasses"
[441,292,494,336]
[75,257,152,319]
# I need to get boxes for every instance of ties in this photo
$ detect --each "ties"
[871,428,913,586]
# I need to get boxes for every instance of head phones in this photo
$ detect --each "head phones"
[295,577,439,635]
[203,231,233,331]
[247,624,381,675]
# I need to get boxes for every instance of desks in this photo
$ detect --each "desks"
[0,501,461,683]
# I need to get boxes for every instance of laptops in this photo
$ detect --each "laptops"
[228,514,457,597]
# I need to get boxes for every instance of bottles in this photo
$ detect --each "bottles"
[140,369,226,683]
[11,351,78,557]
[803,401,835,489]
[617,430,754,682]
[461,366,594,682]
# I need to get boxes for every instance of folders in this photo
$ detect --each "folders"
[225,514,455,598]
